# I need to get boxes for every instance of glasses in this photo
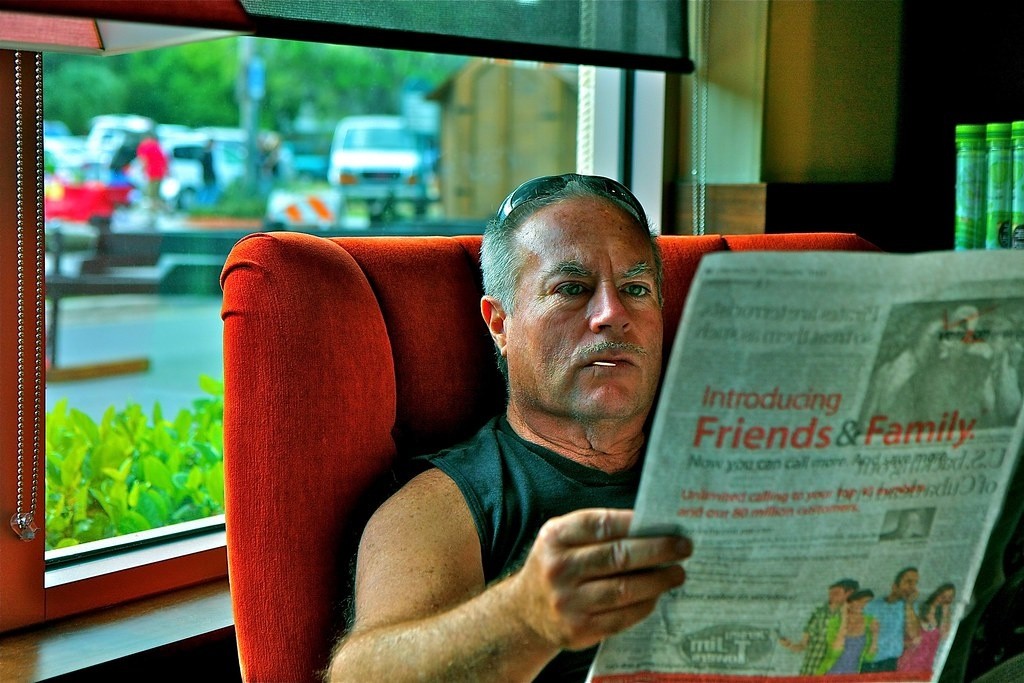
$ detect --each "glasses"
[495,173,651,238]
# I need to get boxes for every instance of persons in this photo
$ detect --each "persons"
[325,173,694,683]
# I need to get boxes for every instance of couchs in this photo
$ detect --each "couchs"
[217,229,875,683]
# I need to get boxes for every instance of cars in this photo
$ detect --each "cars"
[41,113,335,224]
[327,114,429,216]
